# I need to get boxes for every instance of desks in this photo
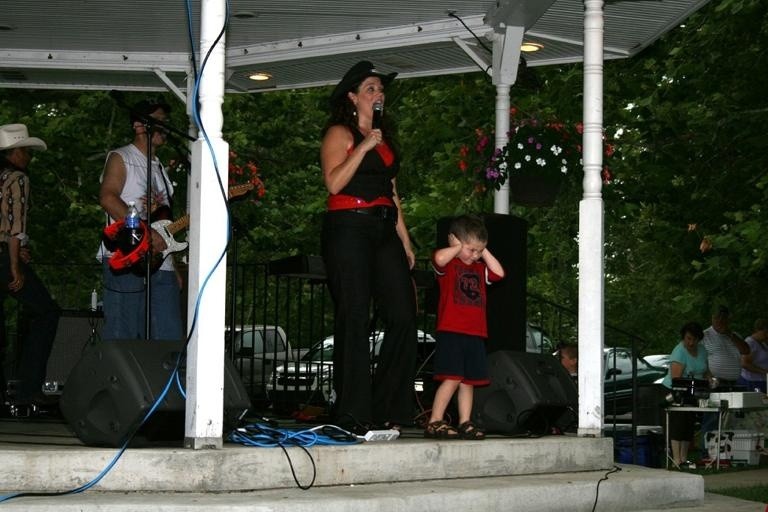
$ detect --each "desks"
[662,403,768,469]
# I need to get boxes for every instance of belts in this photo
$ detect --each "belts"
[350,203,396,221]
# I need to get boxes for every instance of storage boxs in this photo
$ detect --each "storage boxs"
[710,391,763,408]
[701,425,764,465]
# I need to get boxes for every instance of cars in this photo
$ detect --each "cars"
[524,319,555,356]
[643,353,670,368]
[548,342,668,421]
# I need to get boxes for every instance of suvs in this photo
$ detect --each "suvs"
[263,326,438,416]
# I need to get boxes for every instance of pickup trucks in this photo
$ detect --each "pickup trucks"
[225,324,314,412]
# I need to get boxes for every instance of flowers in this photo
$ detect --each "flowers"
[458,106,613,201]
[167,151,264,203]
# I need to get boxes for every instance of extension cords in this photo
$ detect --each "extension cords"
[364,429,400,441]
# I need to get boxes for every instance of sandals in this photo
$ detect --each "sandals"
[456,420,486,439]
[423,419,461,438]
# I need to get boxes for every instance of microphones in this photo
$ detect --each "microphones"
[111,87,127,106]
[370,102,384,143]
[165,131,184,149]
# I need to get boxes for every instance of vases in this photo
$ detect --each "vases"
[507,167,565,212]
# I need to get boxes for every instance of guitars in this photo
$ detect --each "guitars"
[117,179,255,278]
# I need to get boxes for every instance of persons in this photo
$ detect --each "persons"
[736,319,768,417]
[319,61,427,441]
[0,123,62,417]
[423,215,505,440]
[698,305,750,468]
[660,321,720,470]
[96,97,183,341]
[545,343,578,436]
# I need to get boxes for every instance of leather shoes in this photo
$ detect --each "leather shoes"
[14,392,59,408]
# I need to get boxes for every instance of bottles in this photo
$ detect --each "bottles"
[91,289,101,311]
[125,201,139,246]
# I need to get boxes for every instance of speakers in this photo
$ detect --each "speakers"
[56,337,251,446]
[426,213,529,369]
[473,350,577,435]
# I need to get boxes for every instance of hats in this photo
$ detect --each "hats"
[1,123,47,155]
[129,99,171,123]
[330,59,387,105]
